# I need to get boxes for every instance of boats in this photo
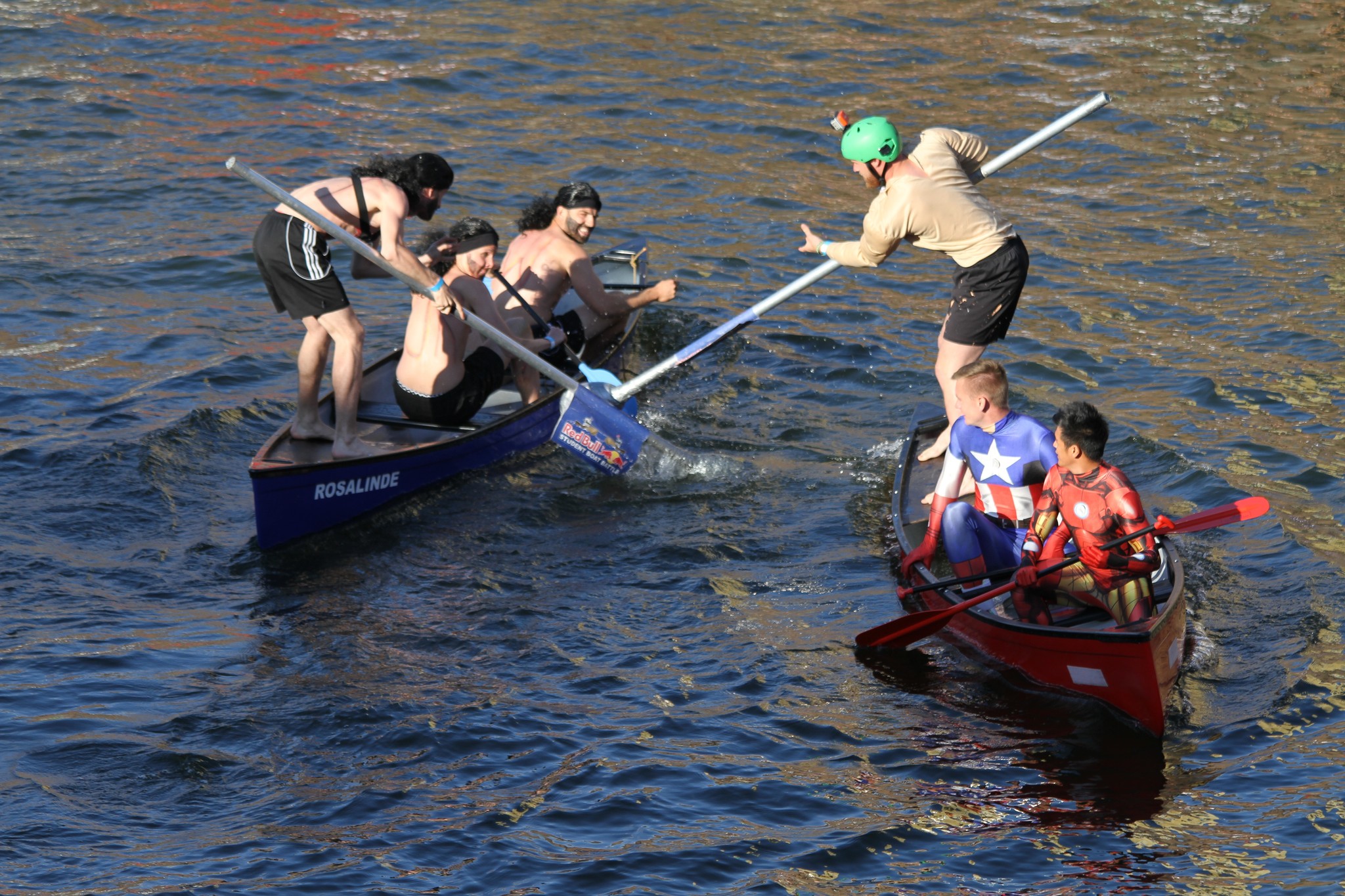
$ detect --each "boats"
[891,400,1188,738]
[247,231,651,554]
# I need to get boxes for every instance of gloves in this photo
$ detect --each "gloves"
[900,545,932,579]
[1036,547,1065,561]
[1015,566,1043,587]
[1079,542,1117,570]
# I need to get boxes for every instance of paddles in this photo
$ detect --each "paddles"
[492,269,638,418]
[569,283,653,292]
[896,494,1270,599]
[854,514,1175,649]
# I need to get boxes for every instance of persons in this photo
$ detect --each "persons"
[392,216,541,427]
[1008,399,1160,627]
[490,181,676,365]
[252,152,466,457]
[799,108,1030,506]
[901,356,1058,596]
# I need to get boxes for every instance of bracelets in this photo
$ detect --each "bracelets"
[543,336,555,350]
[816,240,832,256]
[430,277,444,292]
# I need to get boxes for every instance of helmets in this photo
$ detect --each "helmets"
[841,116,903,163]
